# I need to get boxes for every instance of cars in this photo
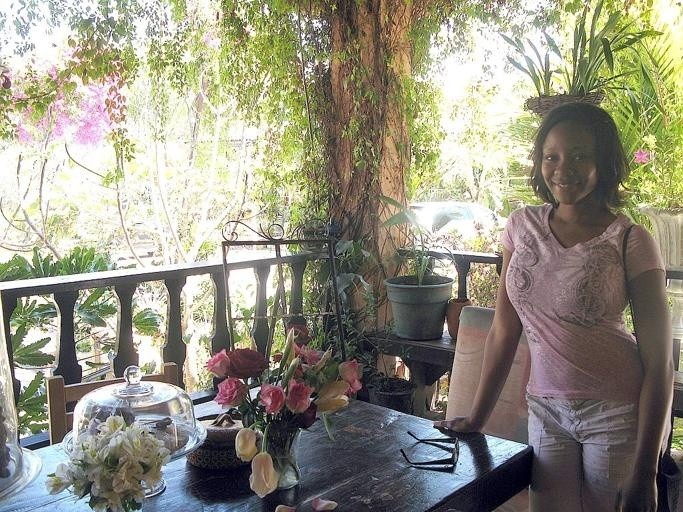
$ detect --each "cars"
[410,201,508,250]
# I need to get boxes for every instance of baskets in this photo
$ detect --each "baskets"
[526,93,605,118]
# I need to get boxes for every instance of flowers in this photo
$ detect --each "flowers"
[635,114,683,208]
[43,410,172,511]
[203,325,363,498]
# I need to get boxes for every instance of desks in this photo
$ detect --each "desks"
[0,378,534,512]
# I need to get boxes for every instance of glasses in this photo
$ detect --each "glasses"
[400,431,460,466]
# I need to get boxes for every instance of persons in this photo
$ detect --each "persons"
[431,101,675,511]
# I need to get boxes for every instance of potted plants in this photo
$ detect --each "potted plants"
[377,194,456,342]
[332,291,418,416]
[500,0,664,119]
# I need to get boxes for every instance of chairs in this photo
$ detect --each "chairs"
[46,362,180,445]
[443,306,532,511]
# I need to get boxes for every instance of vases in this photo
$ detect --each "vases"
[262,423,302,489]
[640,204,682,296]
[139,475,167,500]
